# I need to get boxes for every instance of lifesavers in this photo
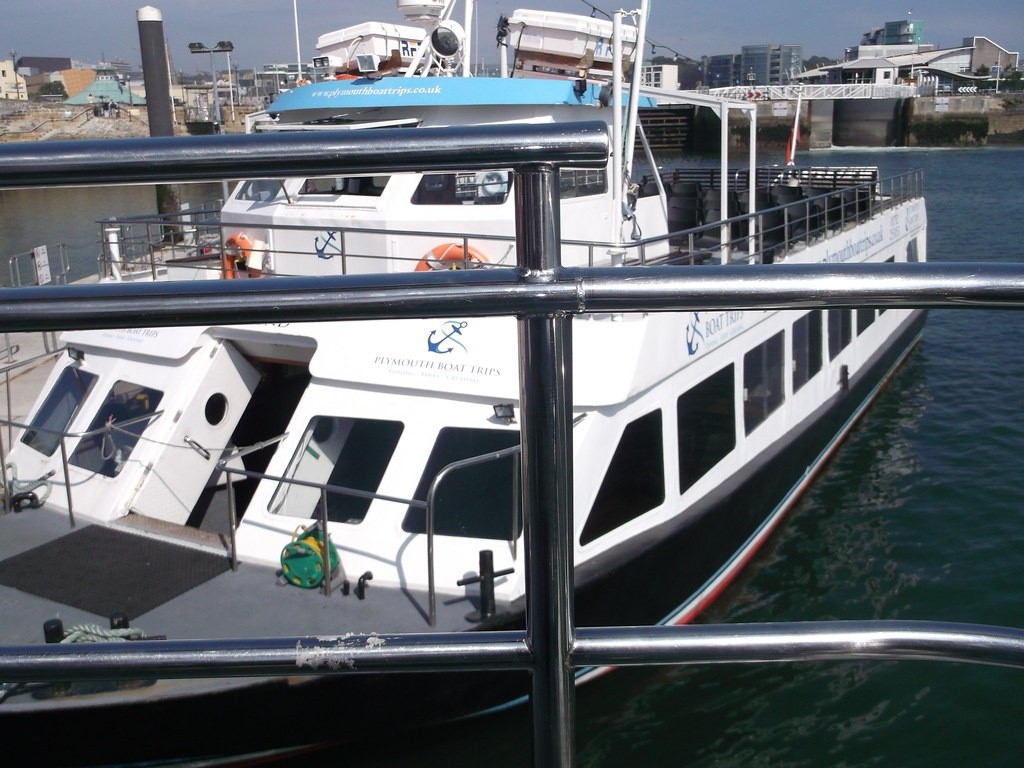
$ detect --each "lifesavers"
[414,244,492,281]
[221,234,262,281]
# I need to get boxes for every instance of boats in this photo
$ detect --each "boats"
[1,1,932,767]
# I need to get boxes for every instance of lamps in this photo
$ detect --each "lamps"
[356,53,381,72]
[313,57,331,67]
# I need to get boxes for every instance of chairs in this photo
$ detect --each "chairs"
[642,182,867,241]
